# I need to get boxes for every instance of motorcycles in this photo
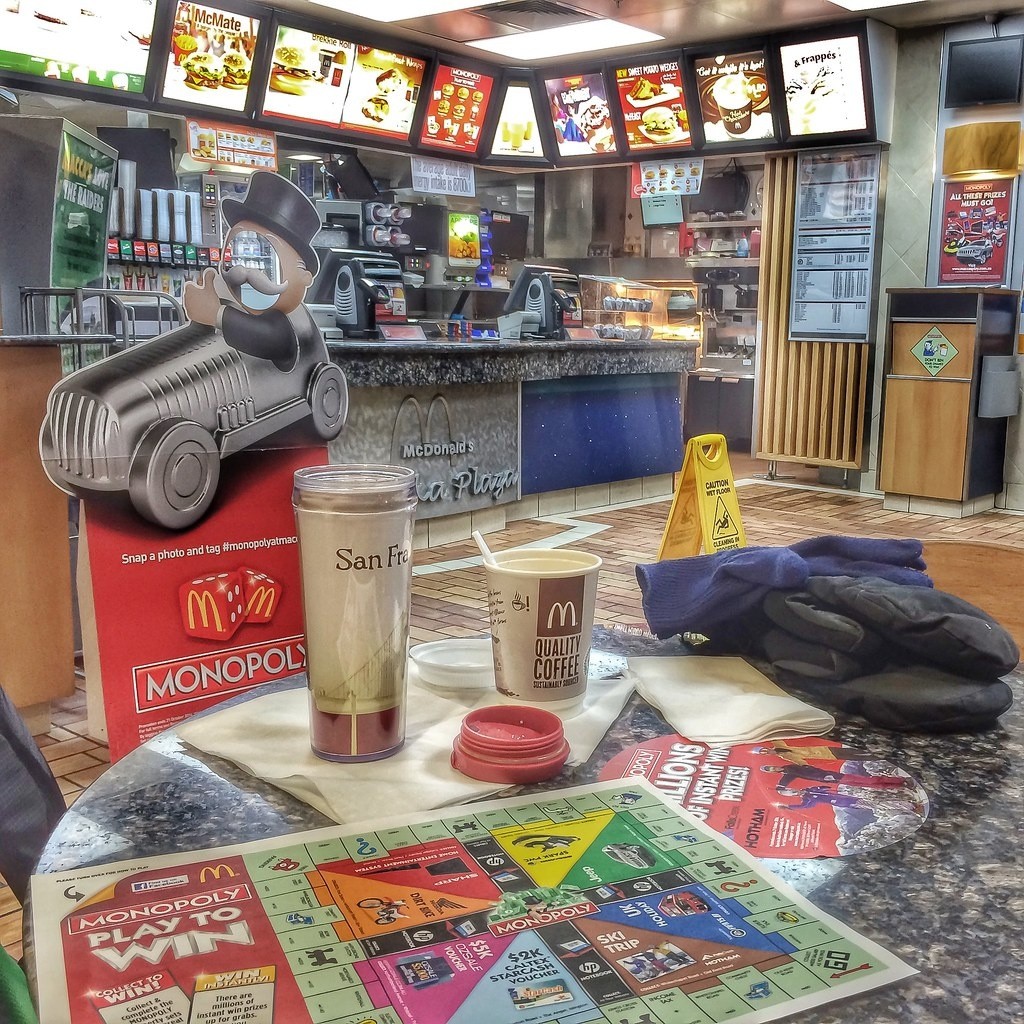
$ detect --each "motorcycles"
[980,228,1007,247]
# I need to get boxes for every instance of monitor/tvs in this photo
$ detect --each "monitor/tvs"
[503,265,569,314]
[689,177,736,214]
[331,152,379,201]
[98,125,177,190]
[944,34,1024,109]
[303,249,393,304]
[640,195,684,229]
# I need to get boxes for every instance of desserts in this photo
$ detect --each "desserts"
[591,297,661,341]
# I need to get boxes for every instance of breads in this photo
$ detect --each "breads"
[192,146,219,159]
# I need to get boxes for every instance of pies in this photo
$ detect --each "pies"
[449,235,481,259]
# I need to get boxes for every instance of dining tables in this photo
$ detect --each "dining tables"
[21,633,1024,1024]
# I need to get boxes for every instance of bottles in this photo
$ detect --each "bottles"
[750,227,761,259]
[737,231,749,258]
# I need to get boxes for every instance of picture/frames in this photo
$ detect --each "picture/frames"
[938,173,1022,288]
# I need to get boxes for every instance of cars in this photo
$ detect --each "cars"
[957,239,993,265]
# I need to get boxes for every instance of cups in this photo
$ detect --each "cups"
[482,547,602,700]
[714,74,753,138]
[292,464,418,764]
[319,49,336,78]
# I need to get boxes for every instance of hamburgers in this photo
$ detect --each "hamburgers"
[645,167,700,179]
[180,49,253,91]
[269,47,313,96]
[642,107,677,135]
[438,83,483,117]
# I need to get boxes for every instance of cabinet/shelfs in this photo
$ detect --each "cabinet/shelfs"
[578,274,655,341]
[683,375,754,453]
[685,220,761,268]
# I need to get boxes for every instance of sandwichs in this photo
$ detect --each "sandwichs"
[630,77,661,99]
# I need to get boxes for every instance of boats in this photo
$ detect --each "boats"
[944,239,972,256]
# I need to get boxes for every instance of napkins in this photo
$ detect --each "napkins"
[386,649,642,765]
[622,651,839,751]
[174,687,514,826]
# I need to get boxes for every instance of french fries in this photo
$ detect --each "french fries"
[175,35,198,50]
